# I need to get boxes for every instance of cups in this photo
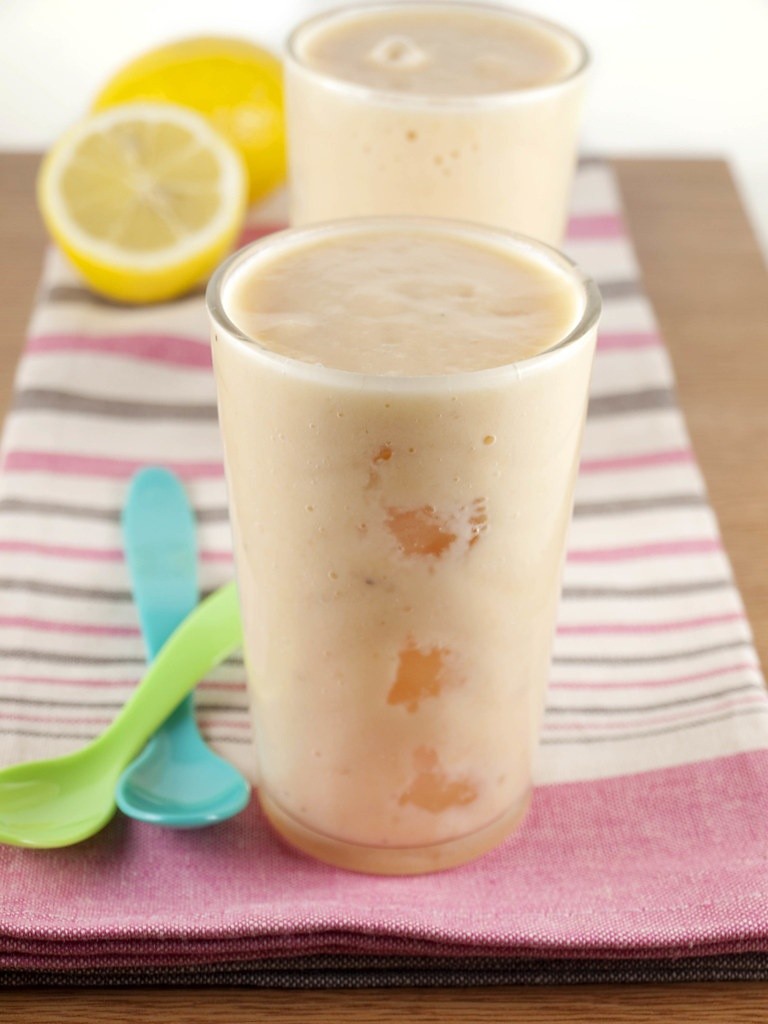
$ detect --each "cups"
[284,0,589,250]
[207,218,604,876]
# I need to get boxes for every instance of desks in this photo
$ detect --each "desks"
[0,152,767,1023]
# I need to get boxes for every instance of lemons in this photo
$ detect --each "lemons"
[38,106,245,301]
[90,35,287,210]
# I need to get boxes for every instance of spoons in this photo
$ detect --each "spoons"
[118,466,251,831]
[0,579,243,851]
